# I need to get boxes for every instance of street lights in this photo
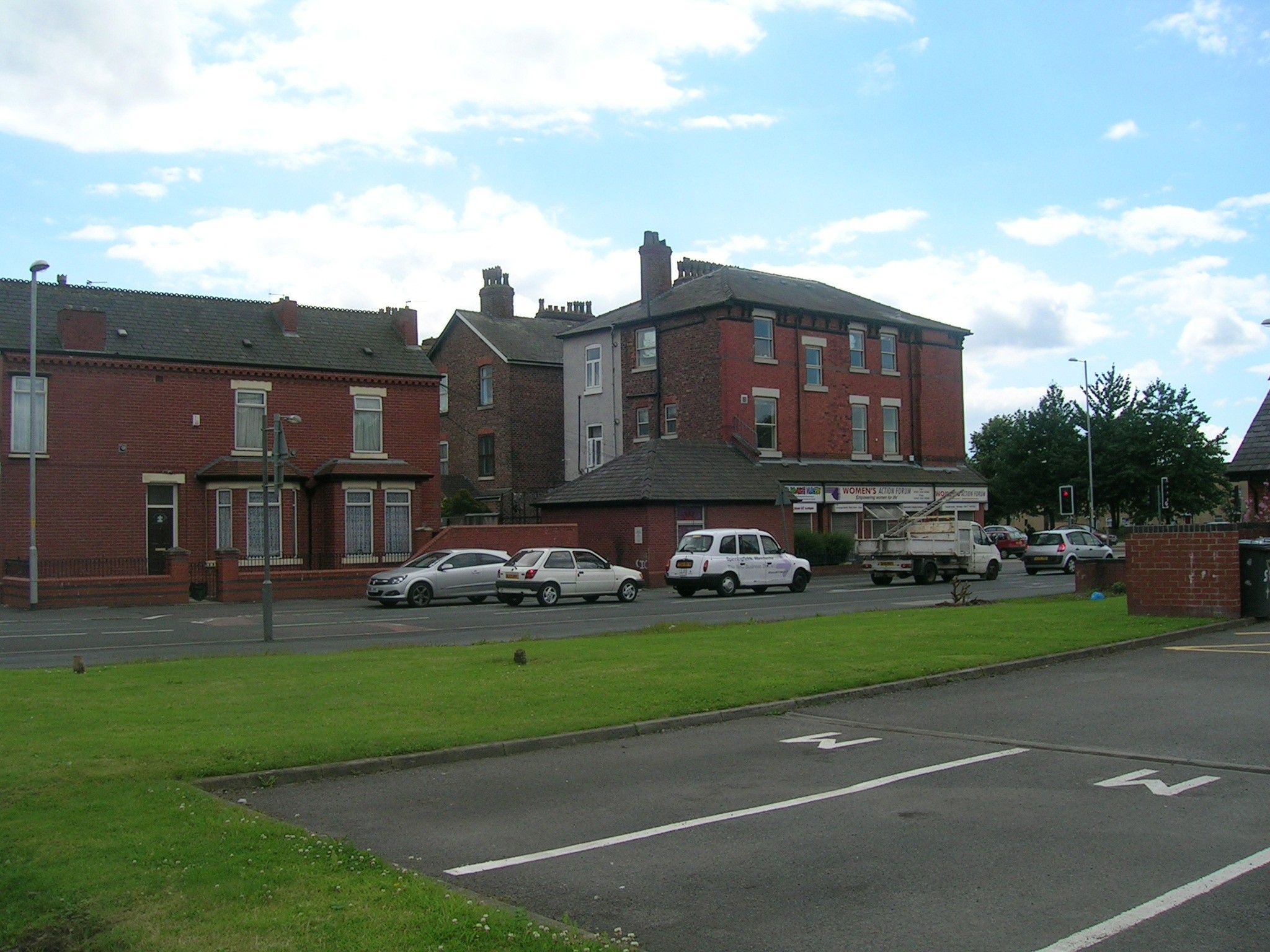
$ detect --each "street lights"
[262,413,303,643]
[1069,358,1094,528]
[30,260,50,611]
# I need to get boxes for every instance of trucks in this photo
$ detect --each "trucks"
[853,510,1002,585]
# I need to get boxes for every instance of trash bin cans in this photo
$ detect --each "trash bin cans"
[1238,537,1270,618]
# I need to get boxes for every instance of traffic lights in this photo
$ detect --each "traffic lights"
[1059,486,1074,515]
[1161,477,1170,508]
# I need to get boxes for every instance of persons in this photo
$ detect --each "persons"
[1025,524,1036,544]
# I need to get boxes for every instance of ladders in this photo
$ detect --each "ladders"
[877,489,963,537]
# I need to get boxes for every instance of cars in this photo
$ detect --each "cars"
[663,527,812,597]
[983,525,1027,539]
[1023,530,1114,575]
[365,548,512,608]
[976,531,1027,559]
[495,547,644,607]
[1037,524,1118,545]
